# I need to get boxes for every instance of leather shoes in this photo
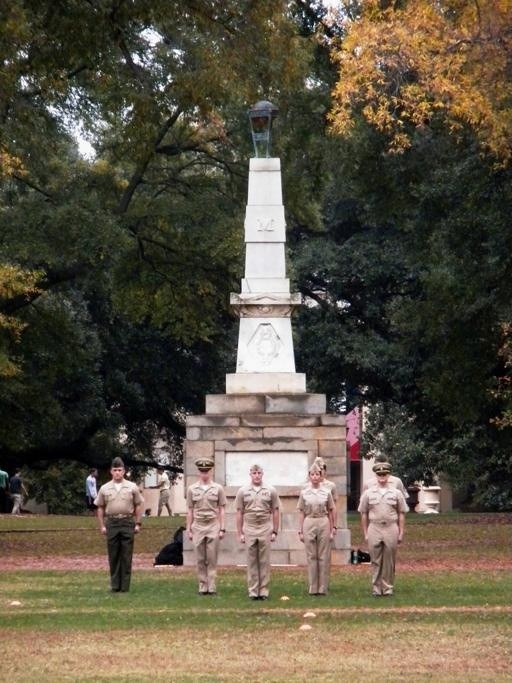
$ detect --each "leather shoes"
[374,593,394,599]
[199,591,216,596]
[250,596,269,601]
[109,588,128,593]
[312,592,328,596]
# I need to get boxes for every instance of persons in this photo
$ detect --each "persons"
[94,457,144,592]
[186,458,227,595]
[156,466,173,517]
[0,467,10,514]
[313,456,337,534]
[358,463,409,597]
[10,469,28,515]
[235,465,280,600]
[364,454,409,503]
[297,464,335,594]
[86,468,98,517]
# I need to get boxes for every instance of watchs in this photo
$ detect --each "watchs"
[273,531,277,535]
[221,530,225,532]
[333,526,337,529]
[136,523,141,526]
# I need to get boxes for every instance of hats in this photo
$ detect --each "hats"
[313,456,326,466]
[372,463,393,475]
[195,457,214,471]
[309,464,320,472]
[112,456,125,467]
[250,464,263,472]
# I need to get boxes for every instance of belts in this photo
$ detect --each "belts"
[110,512,131,519]
[196,519,214,523]
[370,519,395,524]
